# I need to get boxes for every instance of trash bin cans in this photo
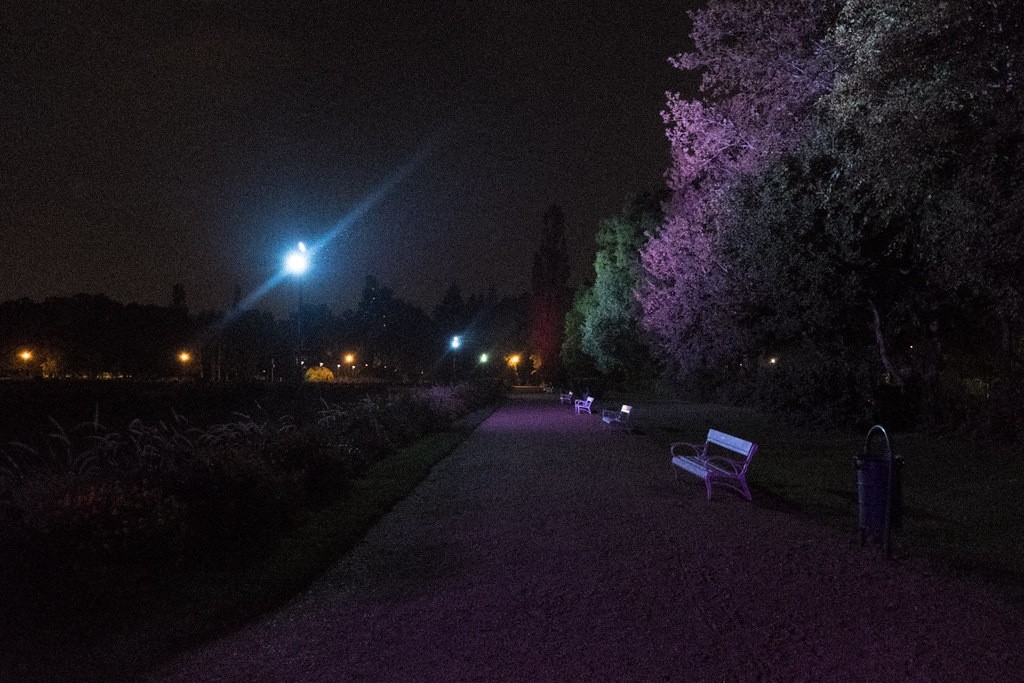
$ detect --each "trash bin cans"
[853,424,903,561]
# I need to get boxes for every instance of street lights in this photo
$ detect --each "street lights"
[284,241,313,385]
[450,335,462,384]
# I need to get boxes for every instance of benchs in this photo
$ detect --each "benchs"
[560,391,573,405]
[546,386,554,394]
[602,405,633,435]
[671,428,758,500]
[575,397,594,415]
